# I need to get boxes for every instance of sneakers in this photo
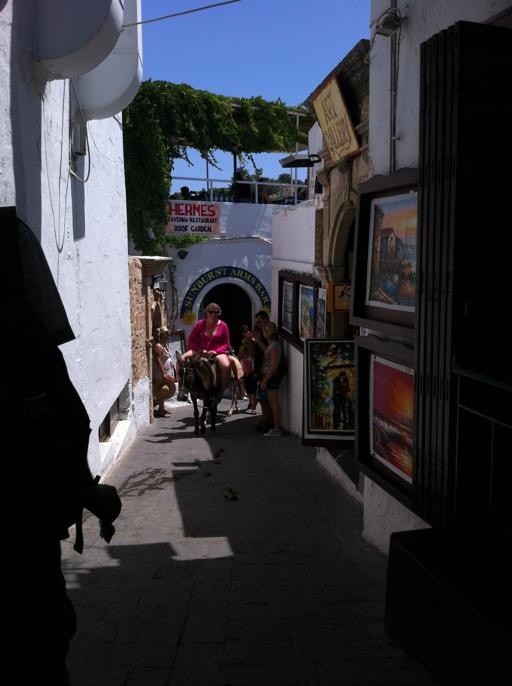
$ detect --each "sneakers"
[240,392,249,400]
[243,406,257,414]
[156,408,172,418]
[253,418,283,438]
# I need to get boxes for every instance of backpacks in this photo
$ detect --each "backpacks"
[0,205,90,554]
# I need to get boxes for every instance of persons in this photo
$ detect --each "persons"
[226,310,286,437]
[0,203,122,686]
[152,324,175,418]
[340,376,352,429]
[331,369,349,429]
[181,185,196,200]
[292,178,307,199]
[251,177,273,203]
[175,301,230,404]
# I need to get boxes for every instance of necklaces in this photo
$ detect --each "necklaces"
[162,342,172,357]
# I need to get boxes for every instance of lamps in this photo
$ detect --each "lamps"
[150,272,167,291]
[279,154,321,168]
[375,7,401,38]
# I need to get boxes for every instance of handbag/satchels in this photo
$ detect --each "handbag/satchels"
[276,356,288,378]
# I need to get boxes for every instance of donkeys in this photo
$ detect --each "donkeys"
[174,348,240,437]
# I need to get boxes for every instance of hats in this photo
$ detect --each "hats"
[152,378,176,400]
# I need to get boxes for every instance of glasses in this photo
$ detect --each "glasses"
[256,319,264,324]
[243,330,247,332]
[207,310,220,315]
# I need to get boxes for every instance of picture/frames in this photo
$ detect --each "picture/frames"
[354,334,419,500]
[159,329,187,384]
[278,269,327,355]
[349,165,421,341]
[304,338,361,449]
